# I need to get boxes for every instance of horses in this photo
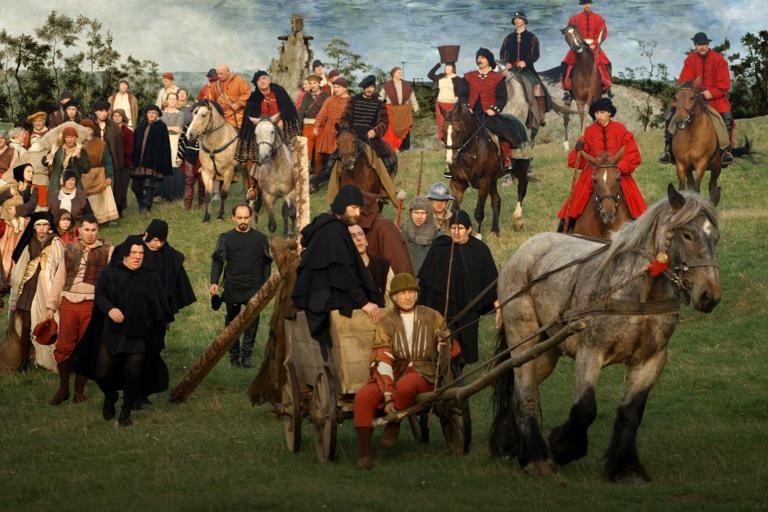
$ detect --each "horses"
[671,76,762,206]
[438,101,541,242]
[334,120,398,215]
[248,111,297,243]
[184,98,245,226]
[562,22,603,154]
[481,182,723,487]
[502,70,586,174]
[573,145,631,242]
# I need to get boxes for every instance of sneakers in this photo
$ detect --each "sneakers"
[152,196,161,204]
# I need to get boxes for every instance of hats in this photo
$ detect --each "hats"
[61,92,126,139]
[163,73,173,80]
[332,78,348,88]
[31,317,59,345]
[388,272,421,297]
[63,170,74,182]
[330,185,364,215]
[254,71,267,85]
[691,32,713,45]
[31,212,54,225]
[589,98,617,120]
[0,126,26,138]
[144,218,168,242]
[511,10,528,25]
[26,111,47,122]
[579,0,593,5]
[425,183,455,201]
[307,59,339,85]
[13,163,31,182]
[118,235,145,257]
[54,209,68,223]
[359,75,375,88]
[207,69,216,77]
[476,47,497,69]
[406,196,437,246]
[449,210,471,229]
[143,105,162,117]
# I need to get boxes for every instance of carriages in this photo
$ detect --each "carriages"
[183,99,329,236]
[276,183,726,486]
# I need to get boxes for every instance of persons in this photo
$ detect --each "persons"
[1,61,464,427]
[459,47,514,173]
[556,97,647,234]
[499,11,546,128]
[352,273,454,469]
[417,210,501,377]
[657,32,734,164]
[560,1,614,104]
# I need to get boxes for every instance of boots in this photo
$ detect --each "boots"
[16,359,30,374]
[659,119,676,165]
[240,315,261,369]
[48,362,71,406]
[357,426,375,468]
[720,118,733,165]
[561,61,568,101]
[99,385,119,420]
[119,399,131,426]
[224,315,241,368]
[500,141,513,171]
[73,374,88,404]
[535,96,546,126]
[182,174,206,211]
[602,63,611,94]
[378,420,401,450]
